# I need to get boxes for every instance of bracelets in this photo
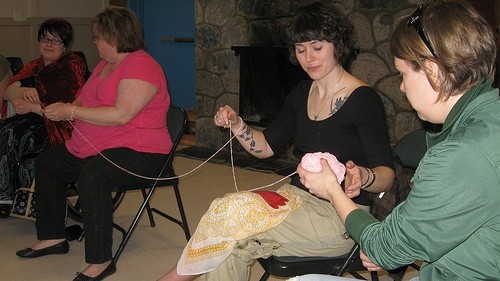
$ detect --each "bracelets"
[360,168,376,190]
[70,105,77,119]
[31,104,34,112]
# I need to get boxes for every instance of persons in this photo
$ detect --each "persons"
[297,0,499,279]
[0,19,89,218]
[157,2,396,281]
[16,6,174,281]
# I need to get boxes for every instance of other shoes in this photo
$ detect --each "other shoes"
[0,208,10,218]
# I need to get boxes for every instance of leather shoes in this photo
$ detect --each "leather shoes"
[16,240,70,258]
[72,259,116,281]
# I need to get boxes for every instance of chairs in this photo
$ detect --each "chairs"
[258,243,379,281]
[78,106,191,261]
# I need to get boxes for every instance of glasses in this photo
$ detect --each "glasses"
[91,35,102,43]
[39,37,64,45]
[406,4,437,60]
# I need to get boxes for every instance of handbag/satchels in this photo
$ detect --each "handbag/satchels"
[9,177,68,224]
